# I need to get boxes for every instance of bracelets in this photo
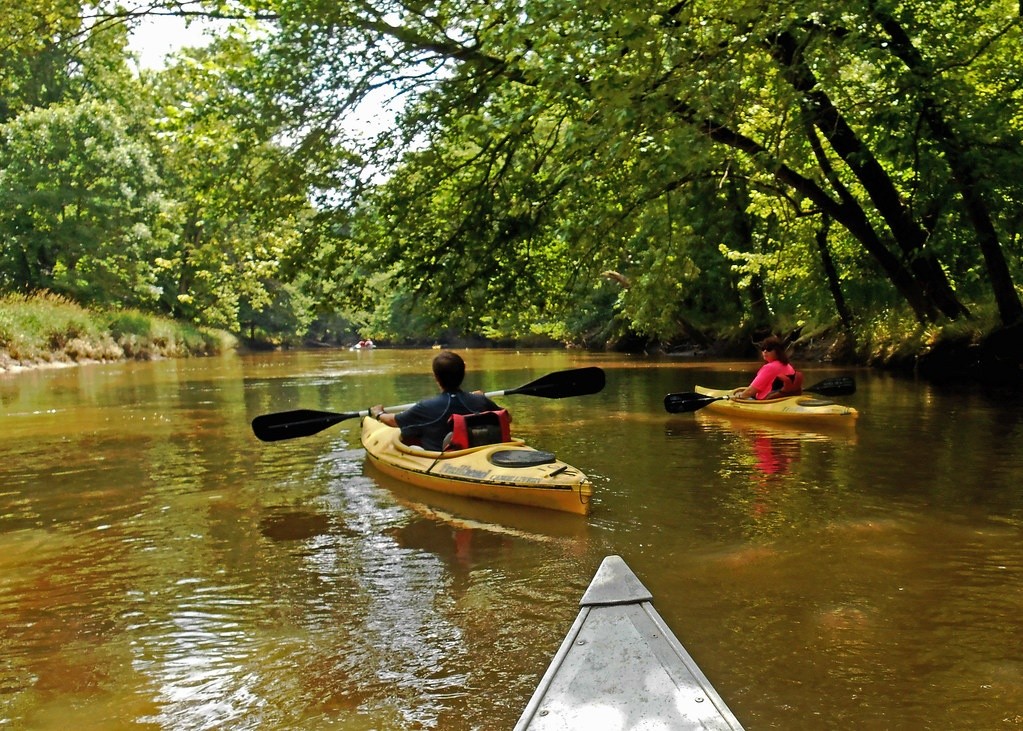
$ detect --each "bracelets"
[376,411,388,421]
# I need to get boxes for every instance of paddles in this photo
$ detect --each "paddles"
[250,365,609,446]
[662,374,858,417]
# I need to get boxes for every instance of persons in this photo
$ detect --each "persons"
[359,339,365,347]
[733,336,797,400]
[370,351,513,452]
[365,338,373,346]
[433,340,438,346]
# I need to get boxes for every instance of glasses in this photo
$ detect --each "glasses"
[760,346,774,352]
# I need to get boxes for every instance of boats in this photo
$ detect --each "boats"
[361,457,587,549]
[695,407,857,447]
[692,385,858,428]
[354,344,377,350]
[360,415,593,517]
